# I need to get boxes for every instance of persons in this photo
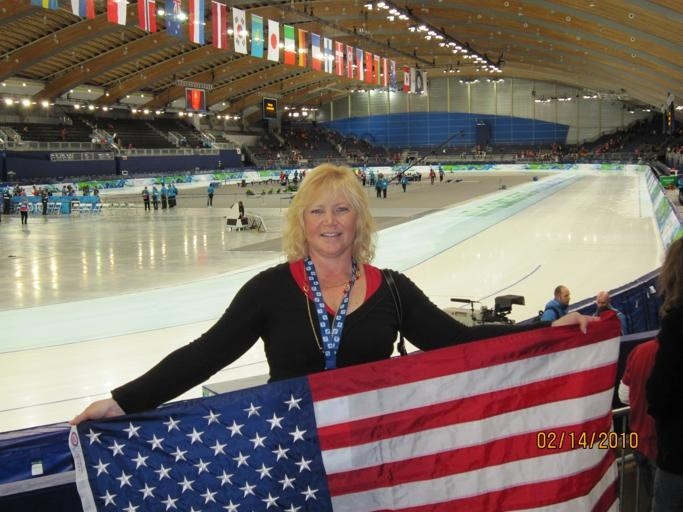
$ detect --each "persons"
[593,291,628,336]
[646,236,683,511]
[618,336,657,504]
[539,285,575,322]
[1,118,682,228]
[68,160,603,425]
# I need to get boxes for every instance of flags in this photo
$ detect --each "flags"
[403,66,424,93]
[32,1,396,91]
[67,310,623,512]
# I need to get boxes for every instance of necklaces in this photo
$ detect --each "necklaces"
[301,257,361,354]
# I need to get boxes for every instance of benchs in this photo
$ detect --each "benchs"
[0,118,682,216]
